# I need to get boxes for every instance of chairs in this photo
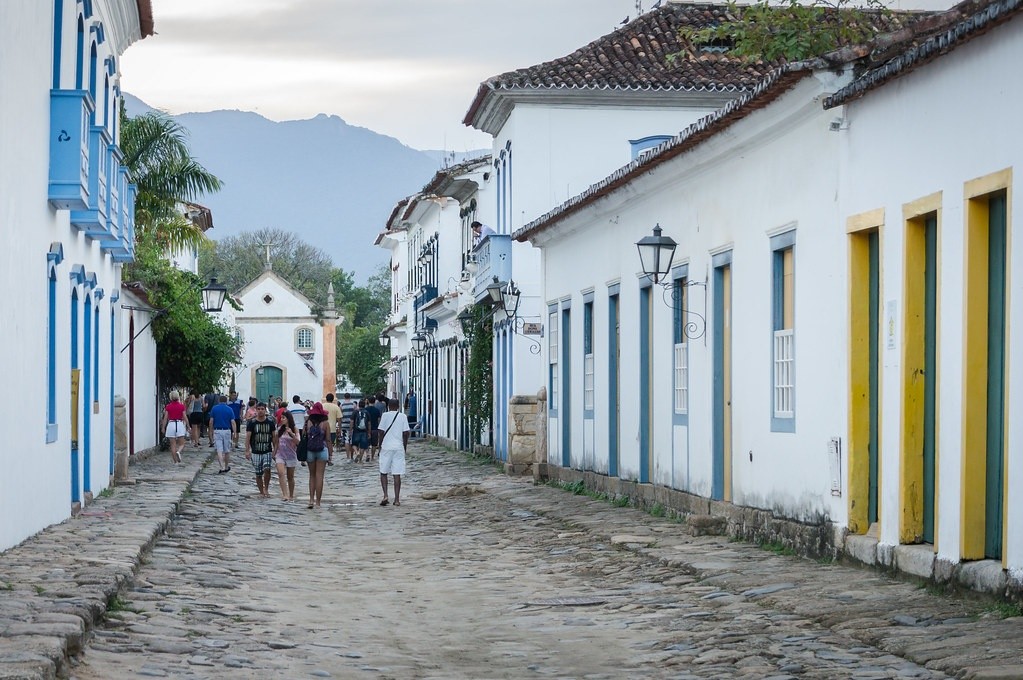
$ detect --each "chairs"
[408,416,424,440]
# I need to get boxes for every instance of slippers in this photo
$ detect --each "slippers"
[380,500,389,505]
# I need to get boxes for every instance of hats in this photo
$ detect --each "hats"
[307,402,330,416]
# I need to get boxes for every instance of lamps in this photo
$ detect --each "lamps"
[411,331,436,352]
[455,275,542,355]
[236,361,264,379]
[121,268,227,354]
[378,333,390,349]
[633,223,708,346]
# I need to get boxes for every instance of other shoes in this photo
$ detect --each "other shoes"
[218,467,230,474]
[327,461,333,466]
[354,453,360,463]
[301,462,306,466]
[175,451,182,462]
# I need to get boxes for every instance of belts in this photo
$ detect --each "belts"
[213,427,229,430]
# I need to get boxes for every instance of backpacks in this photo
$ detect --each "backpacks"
[354,409,368,432]
[306,418,325,452]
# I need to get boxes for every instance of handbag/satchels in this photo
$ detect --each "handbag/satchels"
[295,435,309,461]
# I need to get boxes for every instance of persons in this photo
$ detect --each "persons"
[187,389,206,446]
[302,402,333,509]
[274,411,300,501]
[245,403,276,498]
[246,397,258,421]
[267,395,314,466]
[203,393,220,427]
[377,398,410,506]
[209,396,236,474]
[471,221,496,243]
[322,393,387,466]
[226,391,243,448]
[161,391,191,465]
